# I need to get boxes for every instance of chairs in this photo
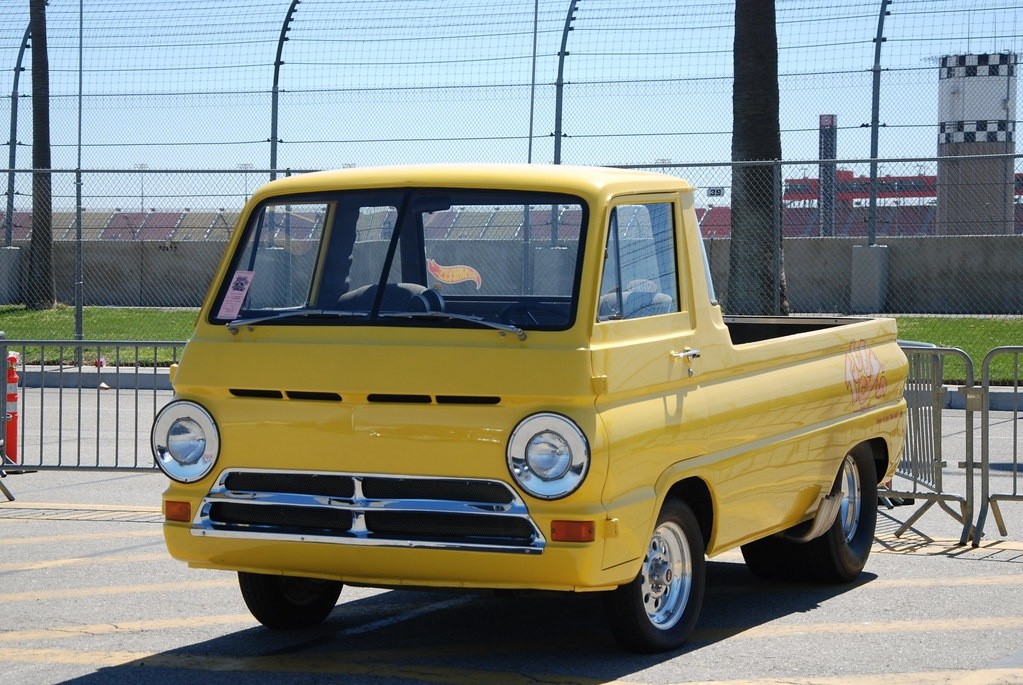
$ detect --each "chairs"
[337,282,428,310]
[599,292,672,321]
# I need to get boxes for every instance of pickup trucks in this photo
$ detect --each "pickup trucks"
[150,161,911,656]
